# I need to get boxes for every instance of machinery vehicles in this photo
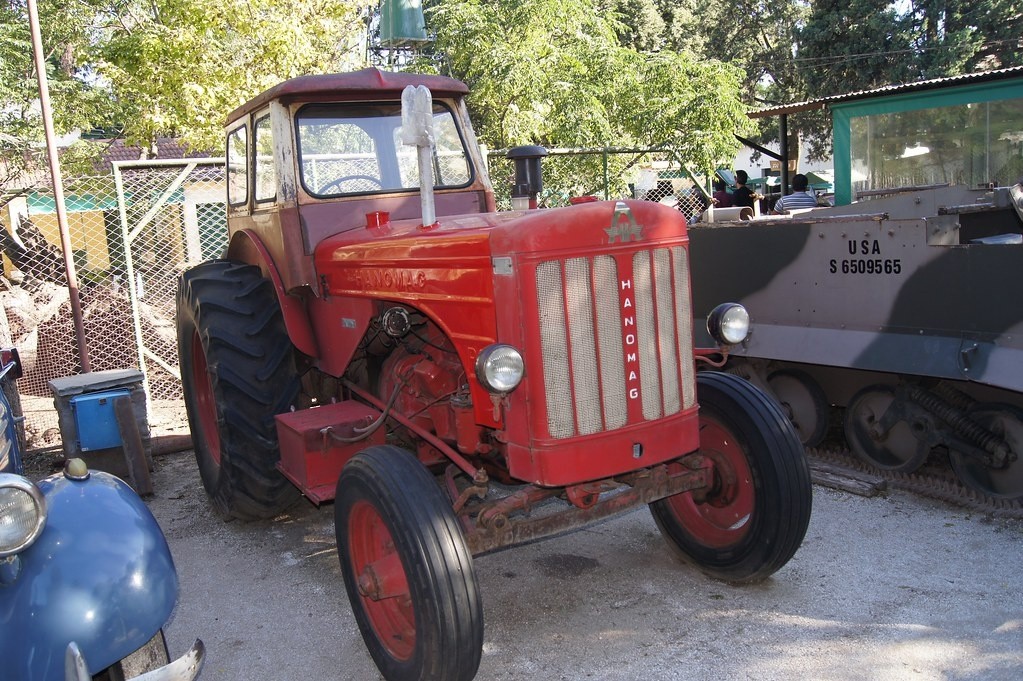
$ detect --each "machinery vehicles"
[682,64,1022,518]
[173,63,815,681]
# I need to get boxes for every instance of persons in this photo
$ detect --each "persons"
[773,174,817,215]
[732,170,756,208]
[713,180,733,208]
[643,180,706,220]
